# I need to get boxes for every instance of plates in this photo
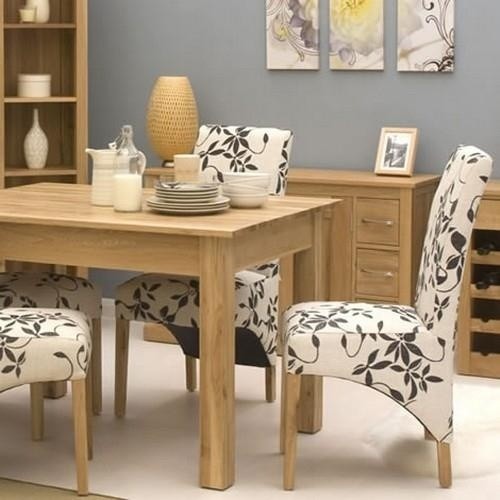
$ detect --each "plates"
[221,172,270,208]
[146,181,231,213]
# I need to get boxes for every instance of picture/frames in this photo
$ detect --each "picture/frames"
[373,126,417,178]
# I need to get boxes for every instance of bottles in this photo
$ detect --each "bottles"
[113,125,142,211]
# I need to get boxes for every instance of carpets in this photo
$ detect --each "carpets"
[0,298,500,498]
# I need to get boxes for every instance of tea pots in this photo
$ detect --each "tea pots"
[85,146,147,206]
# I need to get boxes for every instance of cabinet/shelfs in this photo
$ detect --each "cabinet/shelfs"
[454,182,500,379]
[0,0,89,280]
[143,168,441,344]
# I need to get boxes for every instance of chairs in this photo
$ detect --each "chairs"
[114,123,293,421]
[1,307,93,494]
[1,271,102,441]
[281,144,494,492]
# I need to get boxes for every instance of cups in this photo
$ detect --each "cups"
[173,154,201,182]
[18,7,37,23]
[25,0,50,23]
